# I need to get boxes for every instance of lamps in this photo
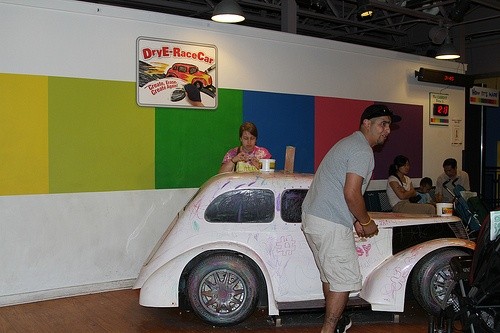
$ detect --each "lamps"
[210,0,246,24]
[434,27,461,60]
[360,7,373,17]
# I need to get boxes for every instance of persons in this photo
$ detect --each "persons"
[301,104,401,333]
[435,158,470,205]
[386,155,437,214]
[219,121,272,173]
[416,177,433,204]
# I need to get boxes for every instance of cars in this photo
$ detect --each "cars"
[133,170,477,328]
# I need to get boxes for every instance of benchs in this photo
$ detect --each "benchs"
[365,186,437,213]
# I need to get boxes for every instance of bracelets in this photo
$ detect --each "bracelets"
[360,215,371,225]
[231,160,236,164]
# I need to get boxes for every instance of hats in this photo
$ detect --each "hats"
[361,104,392,120]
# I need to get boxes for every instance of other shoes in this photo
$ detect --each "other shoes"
[335,317,351,333]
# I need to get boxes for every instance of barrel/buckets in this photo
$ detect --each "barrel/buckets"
[258,158,276,172]
[436,202,453,216]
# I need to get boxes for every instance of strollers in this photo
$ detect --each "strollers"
[429,178,500,333]
